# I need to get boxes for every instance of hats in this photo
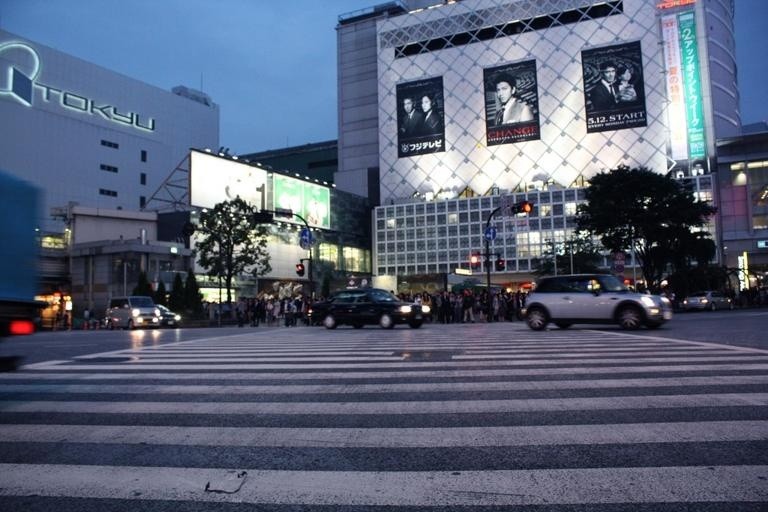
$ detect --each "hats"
[307,288,433,330]
[683,291,735,313]
[154,303,181,328]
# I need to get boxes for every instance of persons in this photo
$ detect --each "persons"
[590,60,624,110]
[397,95,422,138]
[614,66,643,106]
[492,73,534,125]
[418,93,442,135]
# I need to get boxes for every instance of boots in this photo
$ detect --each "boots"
[521,271,673,330]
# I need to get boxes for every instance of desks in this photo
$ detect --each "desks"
[692,167,708,238]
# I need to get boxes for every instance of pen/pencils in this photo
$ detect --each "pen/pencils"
[105,295,161,329]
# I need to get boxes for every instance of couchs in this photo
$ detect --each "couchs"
[471,255,478,269]
[495,260,505,271]
[511,202,531,215]
[295,264,305,276]
